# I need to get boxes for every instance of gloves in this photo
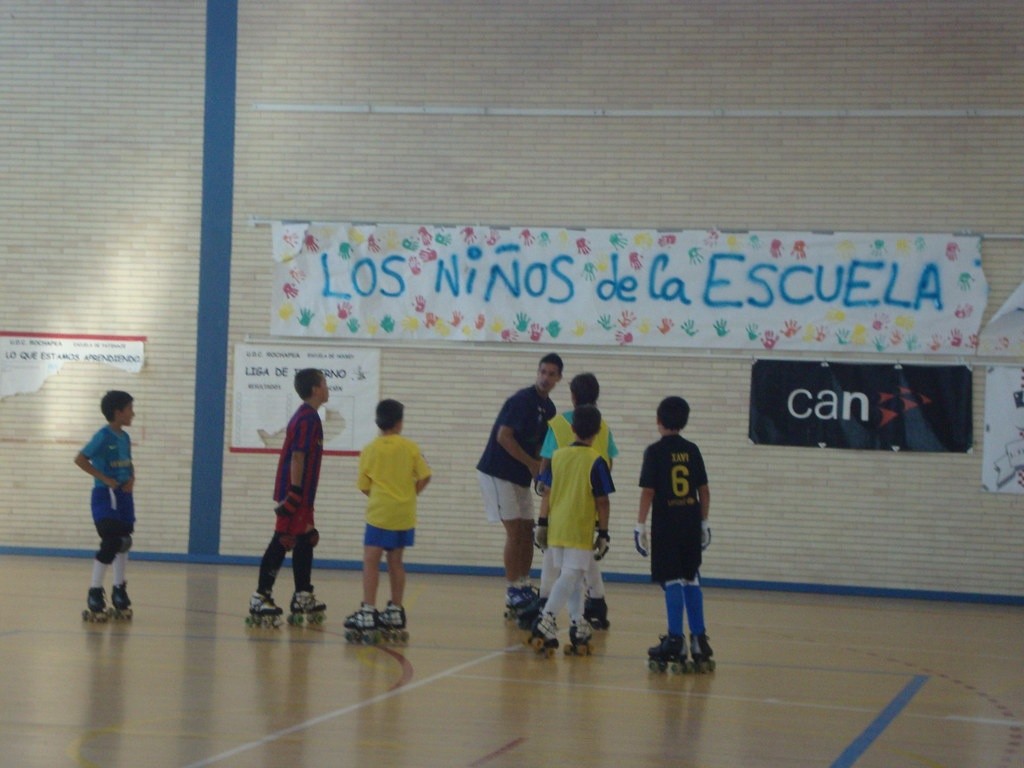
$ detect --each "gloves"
[634,522,650,557]
[593,529,611,560]
[699,519,712,550]
[531,517,548,550]
[274,485,304,517]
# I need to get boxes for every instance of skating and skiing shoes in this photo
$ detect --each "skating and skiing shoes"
[582,594,610,630]
[286,588,327,626]
[563,619,594,657]
[502,583,539,621]
[527,609,560,660]
[106,583,133,621]
[82,585,108,624]
[245,591,284,629]
[688,632,716,674]
[517,594,548,630]
[343,602,383,646]
[647,631,689,674]
[376,601,410,642]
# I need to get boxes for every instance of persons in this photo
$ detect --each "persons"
[527,406,616,655]
[245,368,330,629]
[75,391,136,622]
[344,400,431,641]
[477,353,565,617]
[633,396,716,674]
[519,373,620,630]
